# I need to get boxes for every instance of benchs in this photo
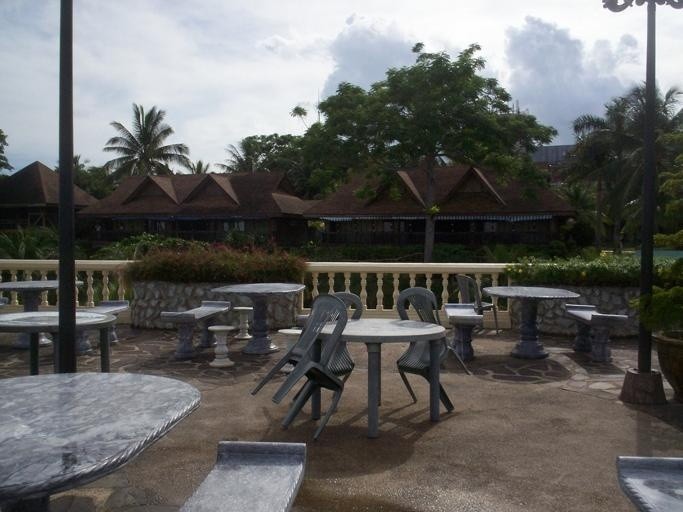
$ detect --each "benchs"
[160,300,232,360]
[615,456,683,512]
[74,301,129,354]
[565,304,628,365]
[444,303,483,371]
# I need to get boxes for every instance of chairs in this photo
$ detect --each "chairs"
[178,440,305,512]
[251,287,455,443]
[456,274,499,337]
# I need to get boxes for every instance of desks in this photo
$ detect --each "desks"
[1,372,202,511]
[211,283,305,355]
[482,286,581,359]
[1,281,85,348]
[0,310,117,376]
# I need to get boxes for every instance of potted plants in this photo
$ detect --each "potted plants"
[629,131,683,403]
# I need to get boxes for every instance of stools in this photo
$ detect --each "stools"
[207,325,237,367]
[231,306,253,339]
